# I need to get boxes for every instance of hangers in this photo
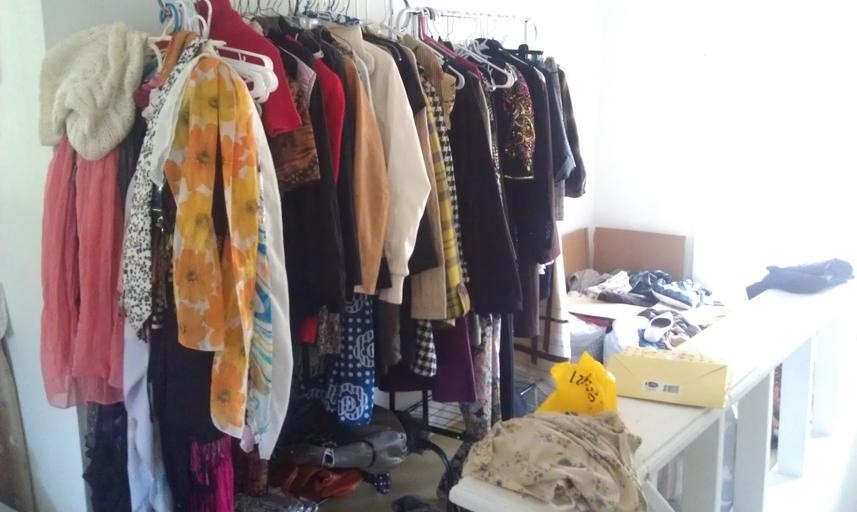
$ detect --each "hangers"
[133,1,279,121]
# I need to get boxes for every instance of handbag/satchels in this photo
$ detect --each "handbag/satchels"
[270,466,362,501]
[651,279,712,310]
[284,404,407,465]
[763,258,852,294]
[606,345,727,408]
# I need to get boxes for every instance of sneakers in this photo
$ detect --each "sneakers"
[643,311,674,343]
